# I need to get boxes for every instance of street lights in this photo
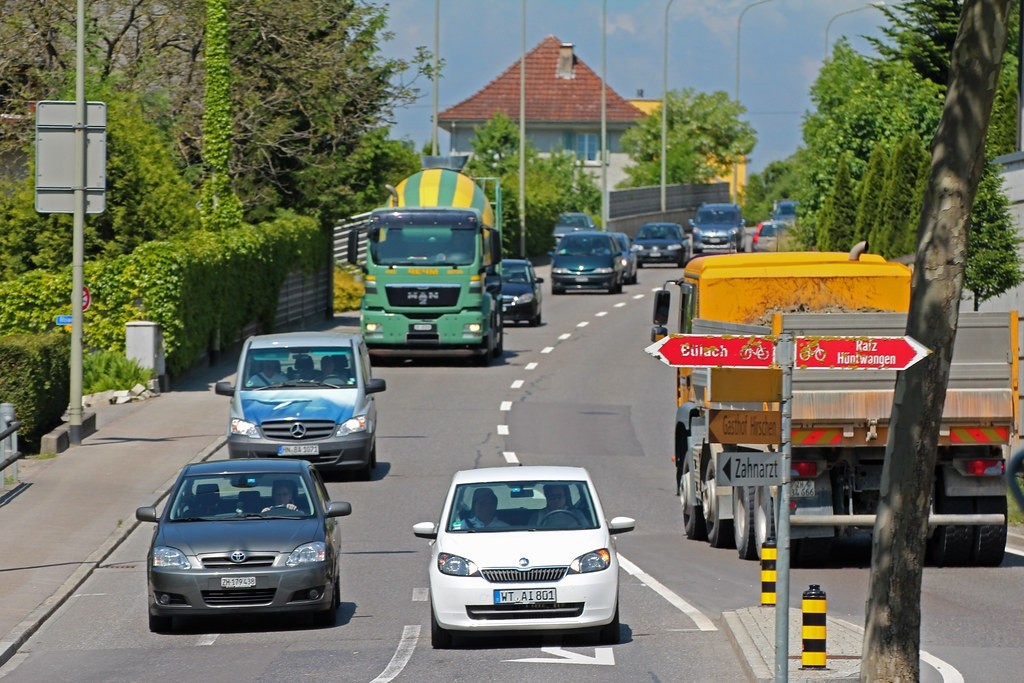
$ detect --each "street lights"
[823,0,889,65]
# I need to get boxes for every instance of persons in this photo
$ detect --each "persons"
[321,356,334,374]
[251,360,288,386]
[462,493,509,528]
[530,485,587,527]
[262,481,298,514]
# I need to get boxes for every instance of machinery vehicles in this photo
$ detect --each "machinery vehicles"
[345,153,503,366]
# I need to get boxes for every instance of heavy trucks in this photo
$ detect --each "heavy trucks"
[649,240,1024,569]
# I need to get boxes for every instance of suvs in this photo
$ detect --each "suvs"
[687,201,746,254]
[547,231,624,296]
[214,332,386,480]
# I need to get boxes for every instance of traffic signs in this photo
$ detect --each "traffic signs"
[714,451,783,487]
[795,333,934,372]
[643,332,776,371]
[704,407,781,446]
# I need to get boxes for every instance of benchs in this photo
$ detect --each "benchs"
[186,491,308,511]
[460,508,591,525]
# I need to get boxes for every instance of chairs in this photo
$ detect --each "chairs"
[701,211,715,222]
[181,484,228,516]
[331,355,355,383]
[285,356,321,380]
[258,360,285,376]
[271,480,310,513]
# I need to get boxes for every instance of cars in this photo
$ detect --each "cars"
[630,221,691,269]
[768,199,801,221]
[609,233,638,285]
[135,457,354,631]
[751,219,792,253]
[412,465,635,650]
[500,258,545,327]
[552,212,596,245]
